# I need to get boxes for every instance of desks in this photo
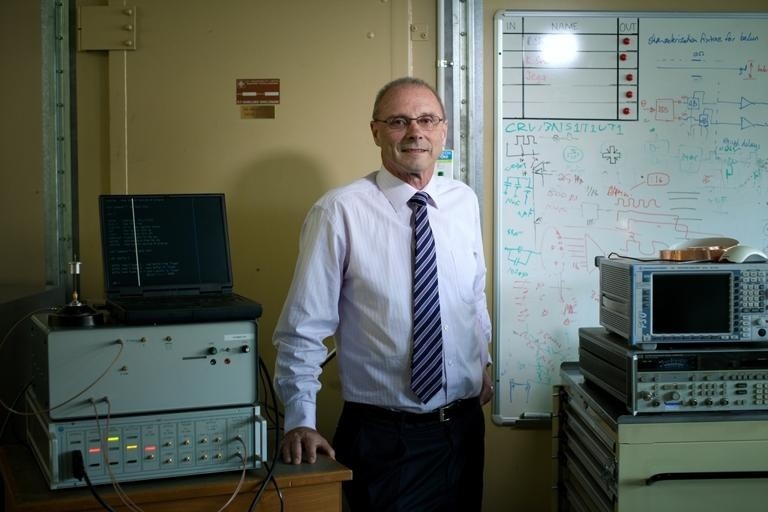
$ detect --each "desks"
[0,422,352,512]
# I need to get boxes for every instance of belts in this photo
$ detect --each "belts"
[344,400,480,422]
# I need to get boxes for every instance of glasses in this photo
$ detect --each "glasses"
[375,114,444,132]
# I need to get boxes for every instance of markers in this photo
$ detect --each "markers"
[520,411,554,419]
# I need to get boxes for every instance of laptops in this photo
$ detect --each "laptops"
[98,192,263,324]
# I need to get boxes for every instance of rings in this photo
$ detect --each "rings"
[489,385,494,390]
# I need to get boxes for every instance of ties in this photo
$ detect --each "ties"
[408,190,443,405]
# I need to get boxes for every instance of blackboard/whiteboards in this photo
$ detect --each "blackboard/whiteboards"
[492,10,768,430]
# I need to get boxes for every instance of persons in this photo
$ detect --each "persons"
[268,74,500,512]
